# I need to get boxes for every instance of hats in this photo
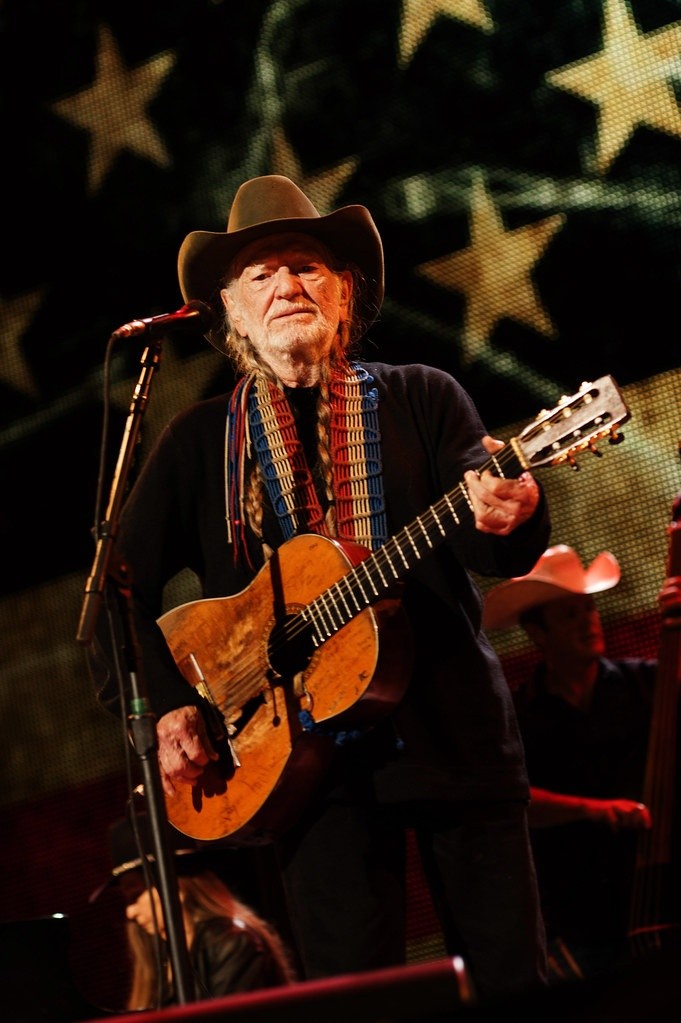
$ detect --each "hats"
[482,543,622,626]
[177,175,385,364]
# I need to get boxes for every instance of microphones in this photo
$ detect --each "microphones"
[112,300,214,343]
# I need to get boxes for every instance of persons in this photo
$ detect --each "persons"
[479,540,681,1023]
[111,813,297,1011]
[86,170,557,1023]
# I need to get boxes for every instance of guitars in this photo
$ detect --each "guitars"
[148,371,636,845]
